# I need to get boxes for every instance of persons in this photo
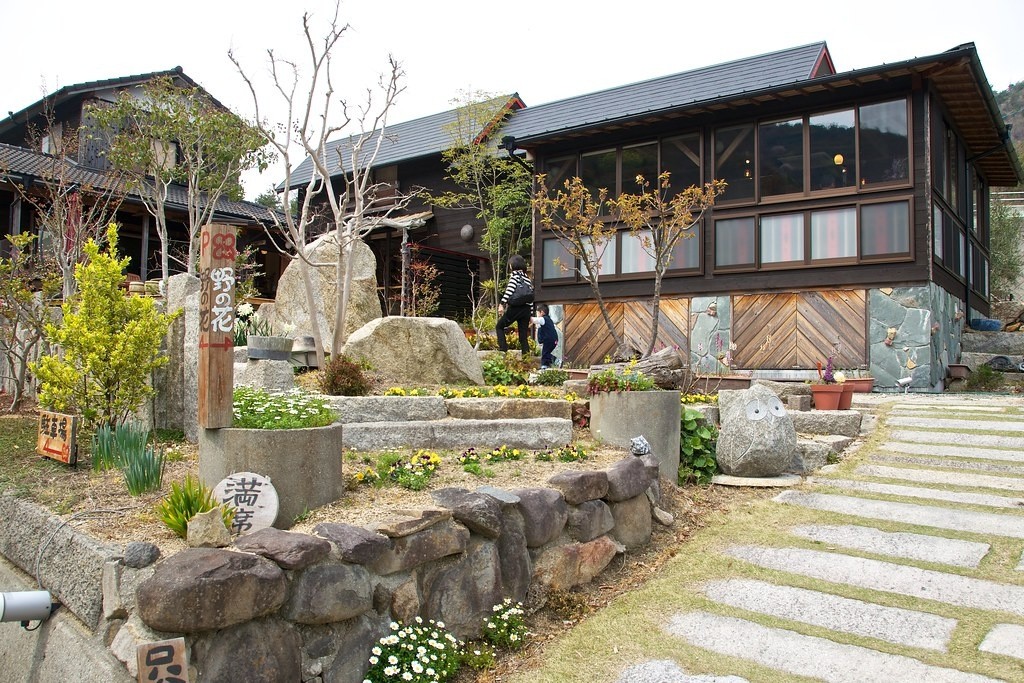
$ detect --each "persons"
[495,254,533,356]
[528,305,563,371]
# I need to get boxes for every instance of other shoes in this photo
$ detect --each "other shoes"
[539,358,561,368]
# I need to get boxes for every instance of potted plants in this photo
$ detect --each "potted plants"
[833,370,855,410]
[198,380,343,530]
[810,356,843,410]
[845,367,876,393]
[583,352,681,487]
